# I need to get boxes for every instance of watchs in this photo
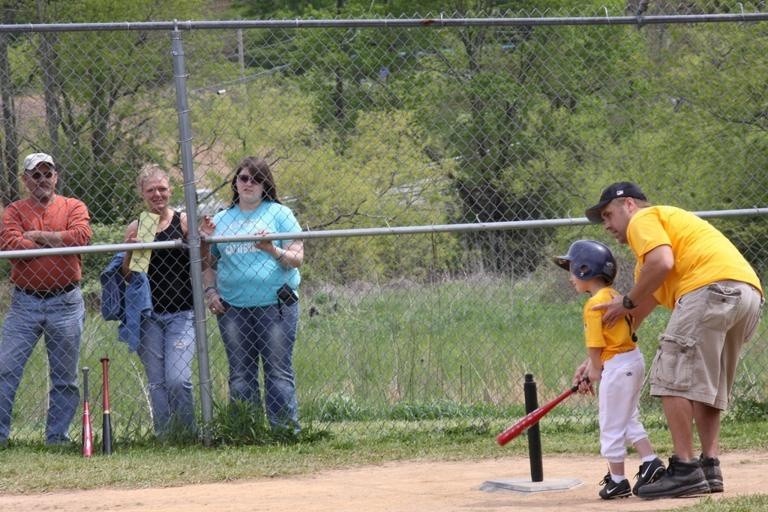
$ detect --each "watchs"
[622,294,639,309]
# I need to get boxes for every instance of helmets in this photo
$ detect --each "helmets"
[552,240,616,286]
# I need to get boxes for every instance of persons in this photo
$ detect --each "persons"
[1,151,92,453]
[121,163,216,444]
[551,241,667,500]
[585,180,764,502]
[204,154,305,440]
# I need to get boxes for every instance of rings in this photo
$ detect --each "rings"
[212,308,216,312]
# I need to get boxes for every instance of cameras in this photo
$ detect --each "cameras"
[277,283,299,307]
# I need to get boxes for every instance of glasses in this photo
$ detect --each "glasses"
[235,174,262,185]
[33,172,53,179]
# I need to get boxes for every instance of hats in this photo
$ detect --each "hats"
[586,182,647,224]
[23,152,55,170]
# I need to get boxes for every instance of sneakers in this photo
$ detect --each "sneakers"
[599,471,632,499]
[637,454,710,501]
[633,456,666,494]
[699,452,724,492]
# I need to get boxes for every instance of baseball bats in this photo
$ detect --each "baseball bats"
[101,358,112,455]
[496,376,590,445]
[82,367,92,456]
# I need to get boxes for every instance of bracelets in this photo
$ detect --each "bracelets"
[205,286,217,293]
[275,248,287,260]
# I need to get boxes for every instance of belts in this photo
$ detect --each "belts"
[15,281,79,299]
